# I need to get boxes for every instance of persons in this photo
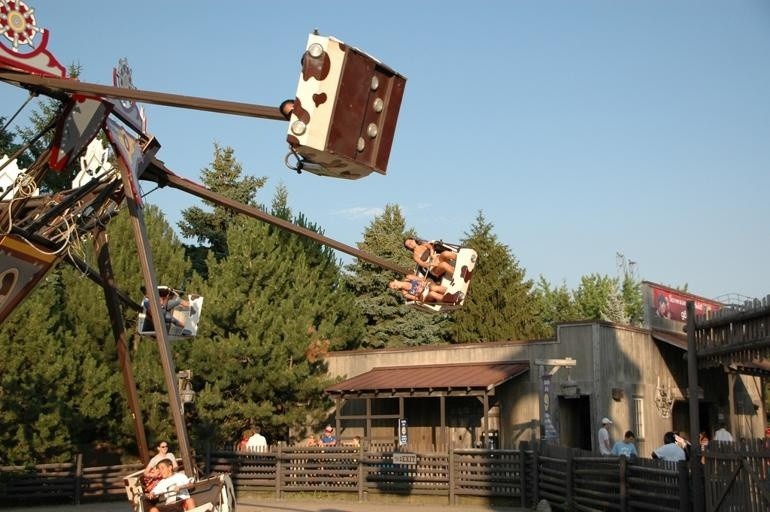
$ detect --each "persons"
[146,459,196,512]
[143,441,178,477]
[351,436,362,448]
[319,426,337,485]
[280,97,299,118]
[596,409,770,485]
[386,274,447,303]
[140,285,191,328]
[247,426,268,454]
[403,236,457,278]
[652,293,672,320]
[240,430,252,451]
[305,433,321,484]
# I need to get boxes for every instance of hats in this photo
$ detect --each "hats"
[602,417,612,424]
